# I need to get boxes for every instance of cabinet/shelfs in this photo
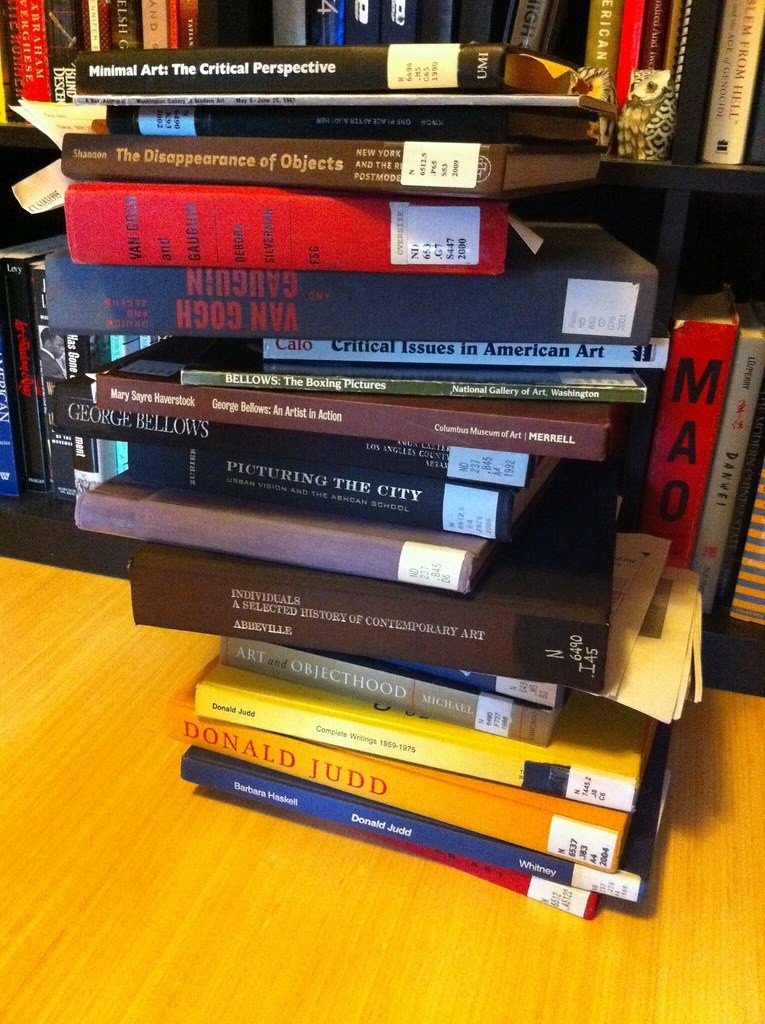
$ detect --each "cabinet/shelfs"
[0,121,765,695]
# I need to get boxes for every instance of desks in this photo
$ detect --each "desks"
[1,557,765,1024]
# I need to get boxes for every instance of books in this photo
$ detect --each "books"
[2,0,765,919]
